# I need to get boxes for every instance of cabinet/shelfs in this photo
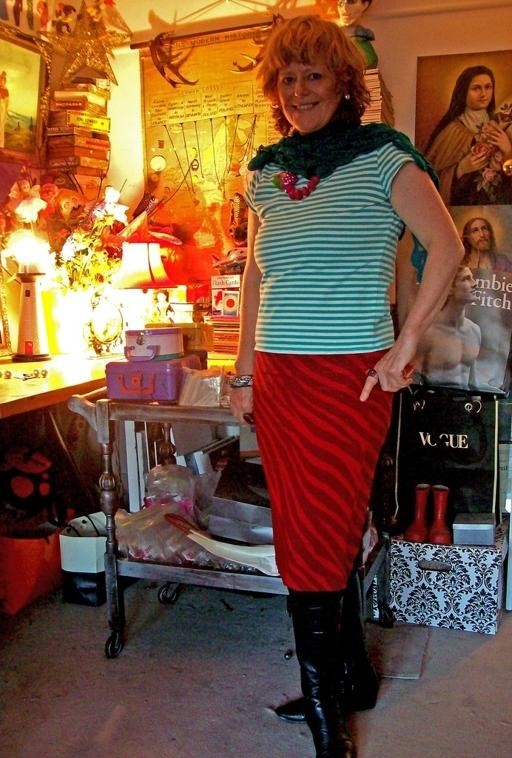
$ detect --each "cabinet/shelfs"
[69,384,384,657]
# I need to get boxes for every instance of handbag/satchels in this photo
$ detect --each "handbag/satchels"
[378,395,501,533]
[59,509,141,608]
[1,508,77,619]
[207,459,274,545]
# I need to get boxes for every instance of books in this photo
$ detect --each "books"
[354,63,396,127]
[204,314,241,370]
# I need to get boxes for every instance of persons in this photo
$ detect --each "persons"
[228,12,466,756]
[460,215,511,272]
[421,63,511,207]
[413,264,482,391]
[335,0,378,68]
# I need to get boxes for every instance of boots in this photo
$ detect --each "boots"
[288,589,359,758]
[403,483,431,543]
[429,485,452,544]
[273,565,378,726]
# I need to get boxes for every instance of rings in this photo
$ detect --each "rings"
[364,366,380,380]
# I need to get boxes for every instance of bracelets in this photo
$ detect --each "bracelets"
[228,375,256,389]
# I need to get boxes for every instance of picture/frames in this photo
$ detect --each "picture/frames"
[0,22,55,163]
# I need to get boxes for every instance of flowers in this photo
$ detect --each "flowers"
[1,164,127,291]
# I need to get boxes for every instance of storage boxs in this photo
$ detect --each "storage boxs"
[105,328,203,401]
[387,525,508,636]
